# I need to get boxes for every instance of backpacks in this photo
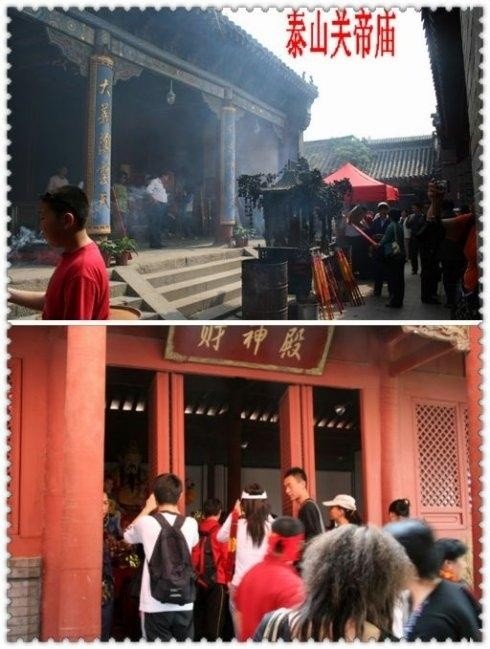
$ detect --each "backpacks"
[197,519,220,594]
[147,515,195,605]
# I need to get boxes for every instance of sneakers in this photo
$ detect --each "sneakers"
[410,269,418,276]
[371,288,456,310]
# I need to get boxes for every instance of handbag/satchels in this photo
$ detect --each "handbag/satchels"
[217,540,235,584]
[388,241,400,255]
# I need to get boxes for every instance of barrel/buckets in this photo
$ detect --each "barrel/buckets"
[290,299,320,320]
[110,305,143,319]
[241,258,290,320]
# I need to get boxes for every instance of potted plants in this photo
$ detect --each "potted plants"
[233,225,257,246]
[99,238,138,267]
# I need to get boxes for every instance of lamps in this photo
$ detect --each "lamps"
[166,79,176,105]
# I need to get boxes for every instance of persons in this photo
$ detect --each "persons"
[6,185,110,320]
[98,467,486,642]
[47,164,169,249]
[331,177,483,320]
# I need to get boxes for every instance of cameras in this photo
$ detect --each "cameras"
[436,180,449,194]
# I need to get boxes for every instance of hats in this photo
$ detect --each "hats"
[321,492,360,511]
[378,200,390,209]
[239,484,269,501]
